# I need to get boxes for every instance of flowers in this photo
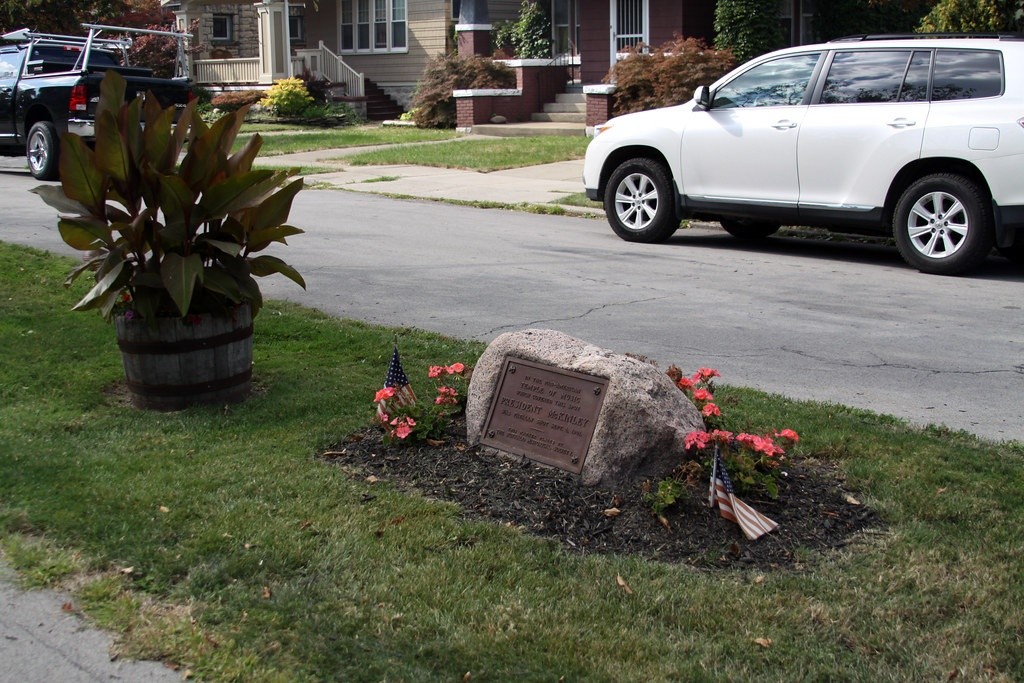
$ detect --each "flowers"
[26,68,306,328]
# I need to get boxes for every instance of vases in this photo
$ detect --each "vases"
[113,299,254,410]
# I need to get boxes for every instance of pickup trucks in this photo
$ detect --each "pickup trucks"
[0,24,201,180]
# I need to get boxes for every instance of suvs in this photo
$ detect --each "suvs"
[581,31,1024,275]
